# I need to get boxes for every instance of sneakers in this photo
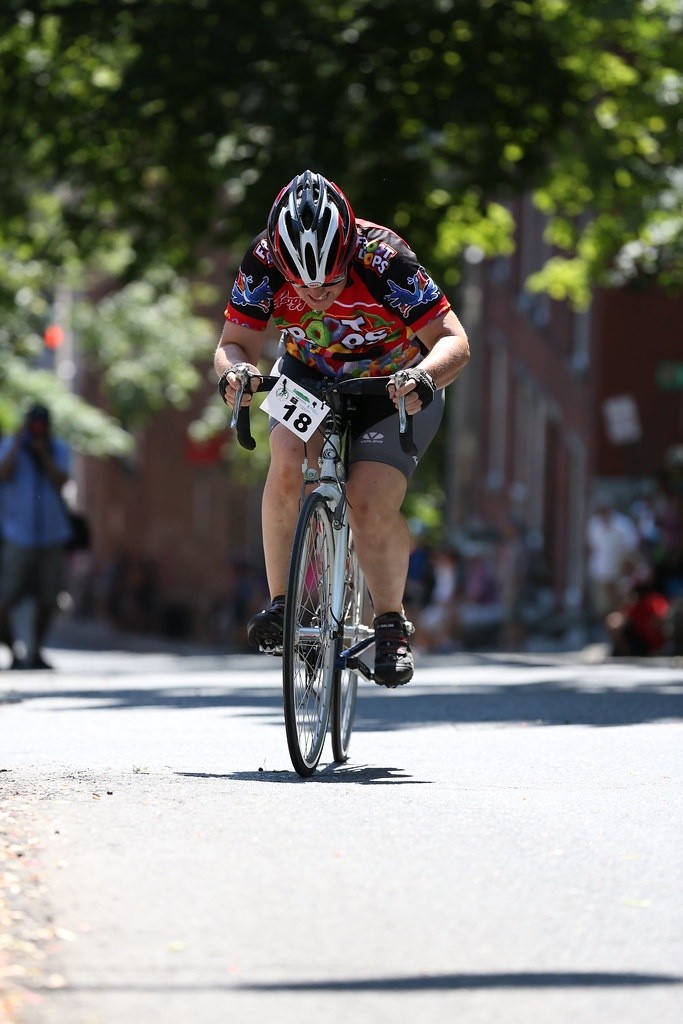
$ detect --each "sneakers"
[247,595,287,653]
[372,608,416,684]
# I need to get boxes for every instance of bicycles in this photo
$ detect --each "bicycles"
[222,359,421,780]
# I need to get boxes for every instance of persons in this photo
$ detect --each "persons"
[584,490,678,658]
[0,404,80,670]
[212,169,471,688]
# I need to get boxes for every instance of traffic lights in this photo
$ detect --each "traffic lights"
[41,322,69,373]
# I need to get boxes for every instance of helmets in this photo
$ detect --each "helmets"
[268,170,359,289]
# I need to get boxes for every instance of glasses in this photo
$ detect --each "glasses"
[284,267,347,287]
